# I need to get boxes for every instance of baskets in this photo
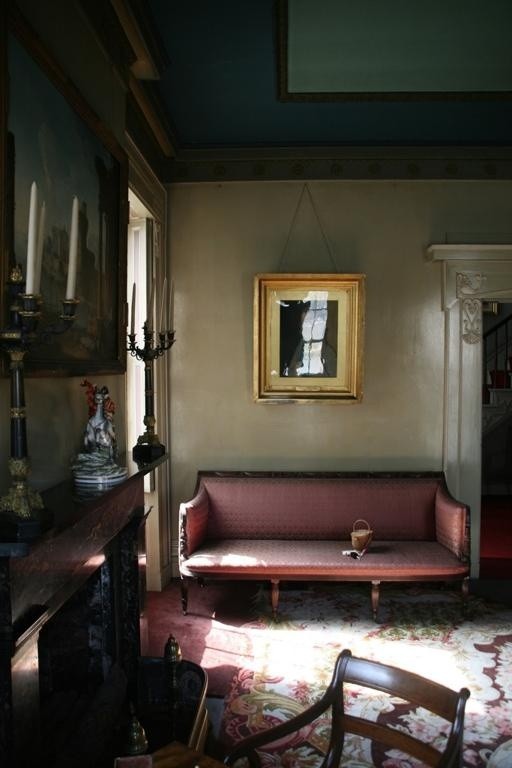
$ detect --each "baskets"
[351,519,373,549]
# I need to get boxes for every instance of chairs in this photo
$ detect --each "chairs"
[220,647,468,768]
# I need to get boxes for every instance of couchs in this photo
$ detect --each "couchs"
[176,470,470,619]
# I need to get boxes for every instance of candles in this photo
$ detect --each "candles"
[24,181,37,297]
[131,283,136,336]
[63,196,83,303]
[168,278,173,332]
[31,200,47,295]
[159,279,167,334]
[146,283,155,332]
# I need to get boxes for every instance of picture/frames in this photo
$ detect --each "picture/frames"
[2,10,129,377]
[251,271,365,403]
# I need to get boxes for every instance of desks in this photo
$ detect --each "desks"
[123,741,231,768]
